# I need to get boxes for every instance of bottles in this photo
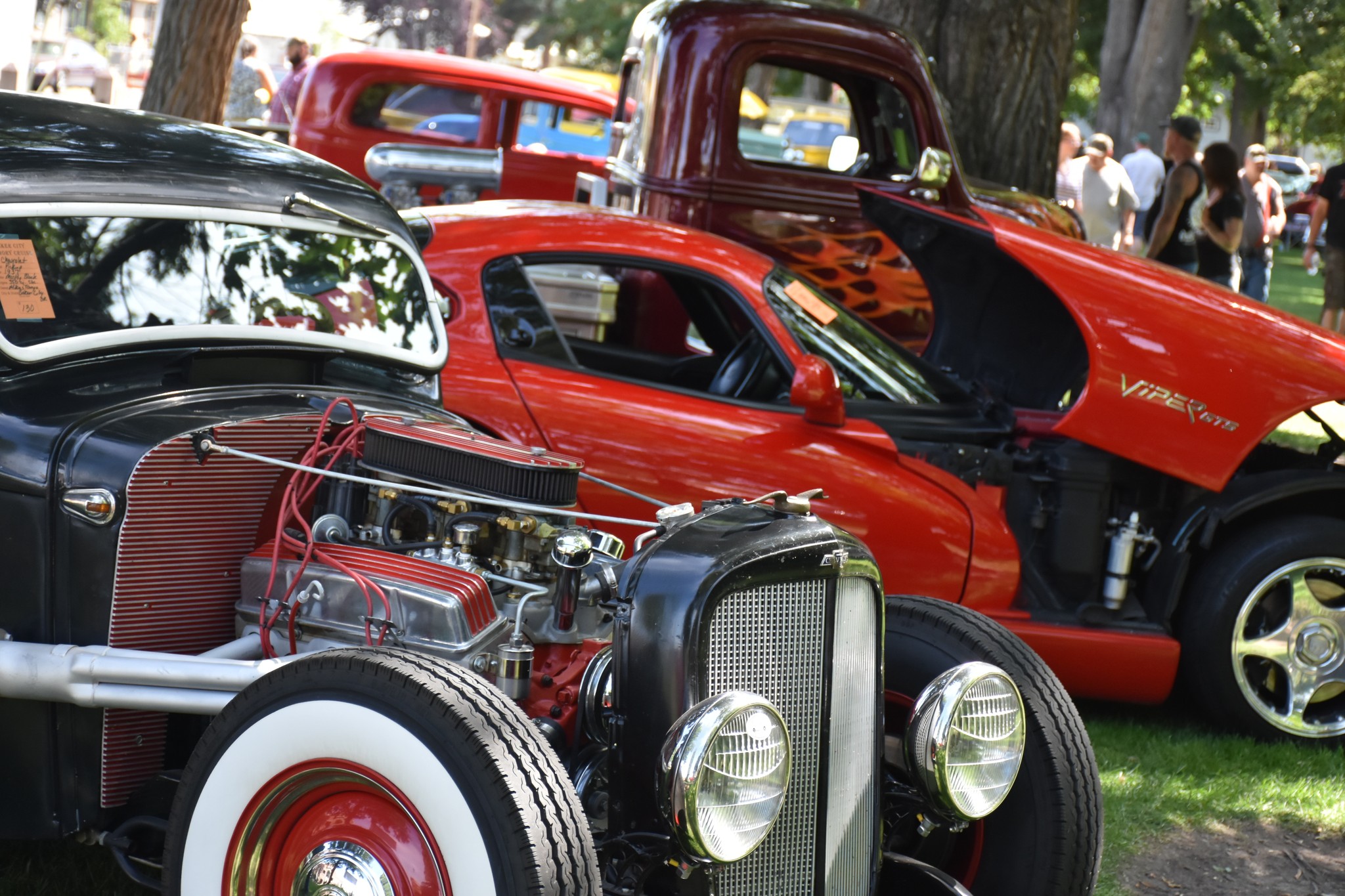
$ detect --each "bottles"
[1306,251,1319,276]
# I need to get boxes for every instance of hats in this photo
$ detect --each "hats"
[1136,132,1151,146]
[1159,117,1202,143]
[1247,143,1269,164]
[1082,133,1114,157]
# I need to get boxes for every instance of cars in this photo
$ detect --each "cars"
[0,92,1111,896]
[1263,154,1328,249]
[0,0,1344,755]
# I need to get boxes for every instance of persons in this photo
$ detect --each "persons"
[1119,128,1162,243]
[1139,116,1210,274]
[1235,144,1285,299]
[1199,139,1241,294]
[1053,113,1141,254]
[222,37,274,129]
[1303,162,1345,334]
[271,35,312,139]
